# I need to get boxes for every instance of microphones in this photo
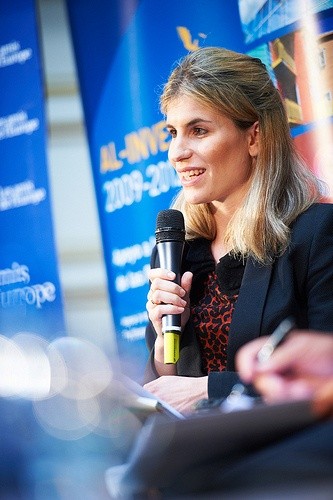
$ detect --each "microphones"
[156,208,186,365]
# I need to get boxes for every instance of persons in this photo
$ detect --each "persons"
[135,48,333,416]
[234,328,332,415]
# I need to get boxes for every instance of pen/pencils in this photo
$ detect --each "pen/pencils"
[225,319,293,412]
